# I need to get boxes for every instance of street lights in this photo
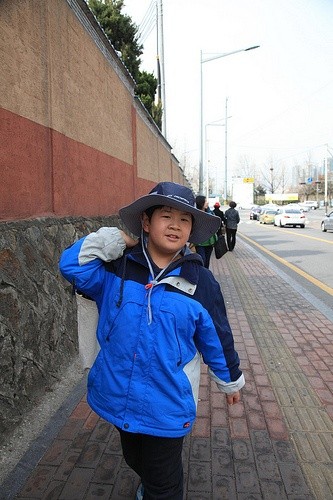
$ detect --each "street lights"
[203,115,232,196]
[198,43,260,196]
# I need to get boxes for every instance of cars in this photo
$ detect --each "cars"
[249,199,333,225]
[321,210,333,232]
[238,202,258,210]
[273,204,306,228]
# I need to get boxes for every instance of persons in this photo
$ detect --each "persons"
[213,202,225,237]
[58,181,245,500]
[225,201,240,251]
[196,196,219,270]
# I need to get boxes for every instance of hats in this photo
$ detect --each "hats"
[215,203,219,206]
[118,182,222,246]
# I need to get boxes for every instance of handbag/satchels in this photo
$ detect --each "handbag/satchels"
[214,236,228,259]
